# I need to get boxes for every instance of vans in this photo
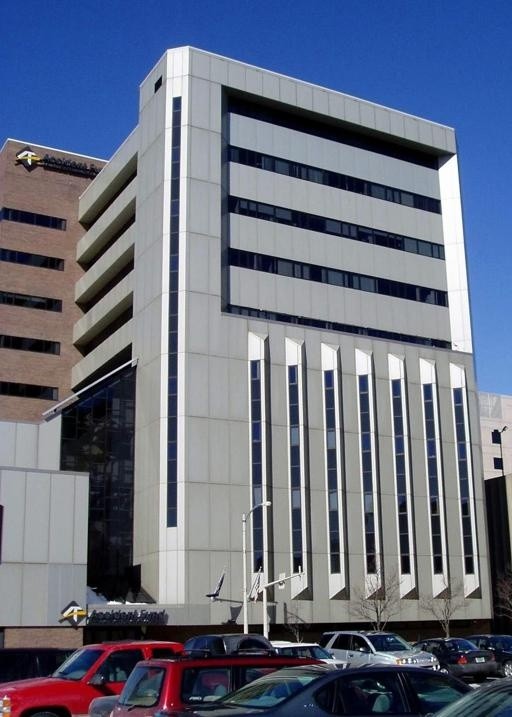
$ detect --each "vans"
[319,630,448,677]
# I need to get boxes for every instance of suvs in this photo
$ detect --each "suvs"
[0,640,184,717]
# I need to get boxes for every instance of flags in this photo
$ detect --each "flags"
[252,571,261,604]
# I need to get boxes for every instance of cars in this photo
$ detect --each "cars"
[86,633,354,714]
[429,674,512,716]
[462,634,511,681]
[409,636,502,684]
[187,663,473,716]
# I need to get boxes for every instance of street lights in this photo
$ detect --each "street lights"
[241,500,272,636]
